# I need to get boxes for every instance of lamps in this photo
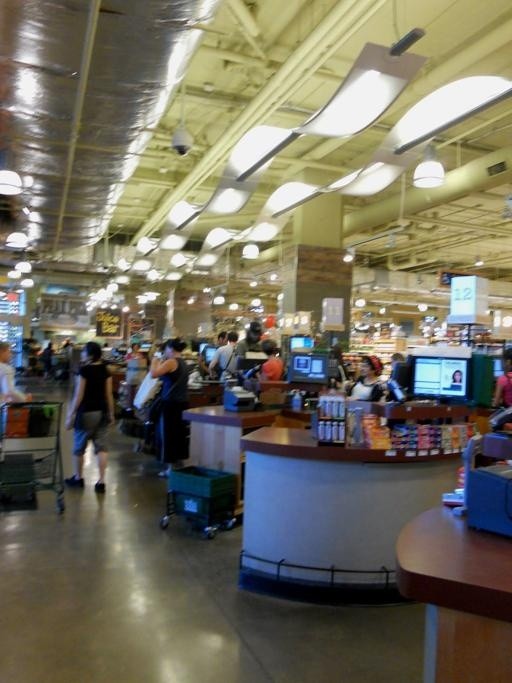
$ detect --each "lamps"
[410,146,446,191]
[0,170,24,197]
[5,233,29,251]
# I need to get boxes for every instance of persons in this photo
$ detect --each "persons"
[125,321,284,392]
[493,349,512,409]
[451,370,462,383]
[64,342,114,492]
[151,336,190,477]
[0,339,114,406]
[345,352,406,431]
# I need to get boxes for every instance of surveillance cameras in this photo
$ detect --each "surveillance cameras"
[172,129,192,156]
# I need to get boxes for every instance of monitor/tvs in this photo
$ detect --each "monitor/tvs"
[289,336,315,354]
[294,356,310,373]
[204,346,219,365]
[311,359,325,373]
[491,355,505,382]
[200,341,207,355]
[411,355,471,403]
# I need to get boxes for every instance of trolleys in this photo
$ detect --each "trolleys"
[1,401,66,515]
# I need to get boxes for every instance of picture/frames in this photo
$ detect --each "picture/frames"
[95,309,125,339]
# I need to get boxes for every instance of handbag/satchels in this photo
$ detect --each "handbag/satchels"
[210,364,223,379]
[135,397,163,426]
[79,410,107,429]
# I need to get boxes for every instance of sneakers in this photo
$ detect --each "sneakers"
[65,475,83,487]
[96,482,105,492]
